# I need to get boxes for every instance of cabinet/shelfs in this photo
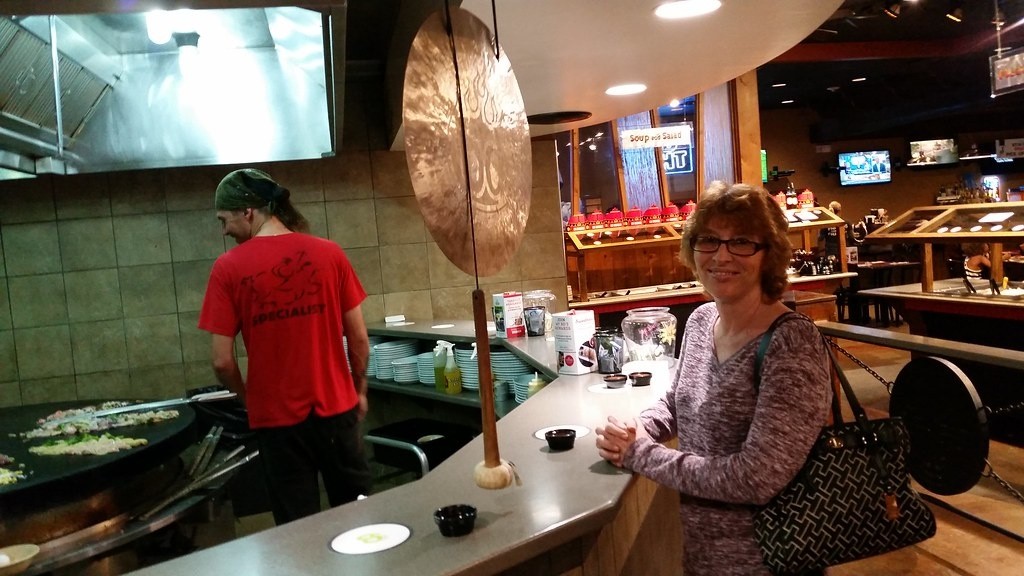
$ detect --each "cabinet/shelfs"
[562,201,859,326]
[857,203,1024,450]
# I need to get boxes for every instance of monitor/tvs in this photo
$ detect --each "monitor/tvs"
[836,149,893,187]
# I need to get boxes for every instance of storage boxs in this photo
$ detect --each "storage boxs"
[491,291,525,338]
[552,310,599,375]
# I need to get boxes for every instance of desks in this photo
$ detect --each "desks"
[851,260,921,327]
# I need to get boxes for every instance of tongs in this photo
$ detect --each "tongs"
[962,279,977,293]
[989,278,1000,295]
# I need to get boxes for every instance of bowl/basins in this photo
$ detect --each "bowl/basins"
[865,215,876,223]
[433,504,477,537]
[545,429,576,450]
[629,372,652,386]
[603,374,627,388]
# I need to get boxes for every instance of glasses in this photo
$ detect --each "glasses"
[690,236,770,257]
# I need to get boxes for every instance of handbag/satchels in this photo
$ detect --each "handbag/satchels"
[751,311,938,576]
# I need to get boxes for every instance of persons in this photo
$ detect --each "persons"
[197,168,371,526]
[595,181,833,576]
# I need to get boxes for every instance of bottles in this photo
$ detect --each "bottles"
[939,182,959,197]
[522,290,556,331]
[528,372,547,398]
[1006,185,1024,202]
[786,182,798,209]
[433,339,448,393]
[621,307,677,369]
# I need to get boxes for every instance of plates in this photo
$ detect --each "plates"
[488,350,553,404]
[342,335,479,390]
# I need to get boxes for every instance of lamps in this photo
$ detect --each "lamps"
[991,9,1007,25]
[946,7,965,23]
[882,0,902,18]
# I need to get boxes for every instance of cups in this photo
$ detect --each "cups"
[494,381,508,401]
[871,208,884,219]
[523,306,545,337]
[593,327,624,373]
